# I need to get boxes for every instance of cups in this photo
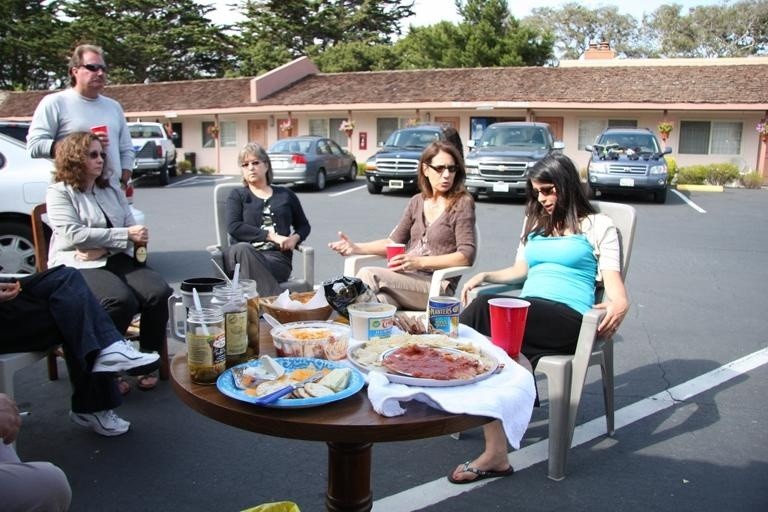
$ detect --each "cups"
[385,243,405,272]
[488,298,531,362]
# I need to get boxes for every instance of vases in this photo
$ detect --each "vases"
[345,131,352,137]
[286,129,291,136]
[761,134,766,141]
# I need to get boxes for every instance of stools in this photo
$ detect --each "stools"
[0,273,65,403]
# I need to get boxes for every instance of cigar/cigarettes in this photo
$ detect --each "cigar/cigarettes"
[15,411,32,417]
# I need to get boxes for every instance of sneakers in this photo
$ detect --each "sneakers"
[68,408,131,437]
[91,339,160,373]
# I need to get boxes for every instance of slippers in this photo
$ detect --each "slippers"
[448,460,515,484]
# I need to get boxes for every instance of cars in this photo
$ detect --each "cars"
[265,135,358,192]
[0,118,146,274]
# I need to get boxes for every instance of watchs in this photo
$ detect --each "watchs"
[118,179,127,189]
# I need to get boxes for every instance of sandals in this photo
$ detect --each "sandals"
[117,374,130,394]
[135,373,158,389]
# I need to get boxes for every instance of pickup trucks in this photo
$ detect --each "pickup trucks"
[126,120,178,186]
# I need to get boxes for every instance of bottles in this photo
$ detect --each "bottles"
[226,278,260,359]
[133,242,148,266]
[185,304,227,385]
[210,284,249,360]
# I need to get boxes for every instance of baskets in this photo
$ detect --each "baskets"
[259,291,332,324]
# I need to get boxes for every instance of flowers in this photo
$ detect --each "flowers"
[281,120,292,131]
[755,121,768,133]
[339,120,355,131]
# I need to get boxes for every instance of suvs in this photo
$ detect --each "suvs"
[363,126,462,195]
[586,127,672,204]
[463,122,566,202]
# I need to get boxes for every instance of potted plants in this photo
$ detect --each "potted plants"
[208,126,218,138]
[659,122,672,140]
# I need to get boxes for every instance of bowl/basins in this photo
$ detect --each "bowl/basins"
[347,302,396,341]
[270,321,351,361]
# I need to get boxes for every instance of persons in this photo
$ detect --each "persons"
[328,142,477,309]
[447,153,629,484]
[46,131,170,395]
[0,392,72,512]
[0,265,159,437]
[27,45,134,192]
[224,141,312,297]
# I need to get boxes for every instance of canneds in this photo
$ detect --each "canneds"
[428,295,461,338]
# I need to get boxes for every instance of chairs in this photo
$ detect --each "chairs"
[451,200,637,481]
[205,183,314,292]
[343,222,481,331]
[30,202,66,381]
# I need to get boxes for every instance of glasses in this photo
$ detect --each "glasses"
[90,150,107,159]
[241,159,265,167]
[422,160,459,173]
[80,63,106,72]
[531,185,555,197]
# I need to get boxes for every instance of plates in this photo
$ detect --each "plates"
[217,357,365,409]
[347,333,500,387]
[380,345,484,379]
